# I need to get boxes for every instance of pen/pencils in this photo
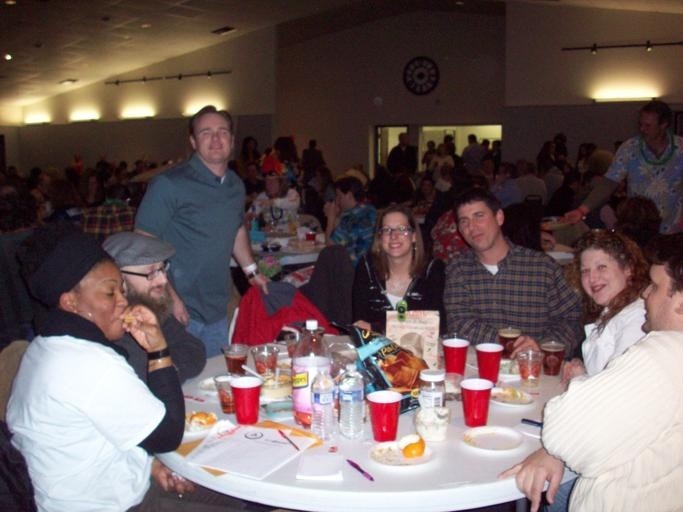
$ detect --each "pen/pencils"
[347,459,374,482]
[278,429,300,451]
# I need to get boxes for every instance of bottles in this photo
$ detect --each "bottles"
[419,369,444,408]
[293,320,331,429]
[339,363,363,438]
[311,365,337,442]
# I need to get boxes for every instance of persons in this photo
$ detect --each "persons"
[5,239,193,512]
[352,206,447,343]
[326,180,378,266]
[246,173,299,229]
[499,252,683,512]
[1,161,136,239]
[445,192,586,359]
[234,137,260,176]
[564,230,649,391]
[562,101,683,239]
[613,196,671,260]
[136,105,273,358]
[101,232,208,384]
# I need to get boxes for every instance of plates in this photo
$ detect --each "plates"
[199,377,218,396]
[462,426,526,453]
[490,387,536,408]
[366,441,435,468]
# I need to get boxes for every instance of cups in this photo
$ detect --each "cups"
[367,390,402,443]
[519,352,541,389]
[221,344,248,376]
[443,338,470,376]
[475,343,504,384]
[498,328,520,361]
[232,376,260,426]
[460,378,493,427]
[285,333,310,359]
[302,327,323,355]
[250,346,279,374]
[214,374,233,413]
[543,343,564,376]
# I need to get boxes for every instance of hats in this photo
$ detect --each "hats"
[15,211,106,308]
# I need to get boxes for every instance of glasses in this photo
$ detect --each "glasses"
[120,261,171,280]
[380,225,412,236]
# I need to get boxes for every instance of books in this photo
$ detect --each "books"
[186,422,319,476]
[385,310,440,369]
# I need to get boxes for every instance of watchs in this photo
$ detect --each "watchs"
[147,347,168,362]
[246,268,260,282]
